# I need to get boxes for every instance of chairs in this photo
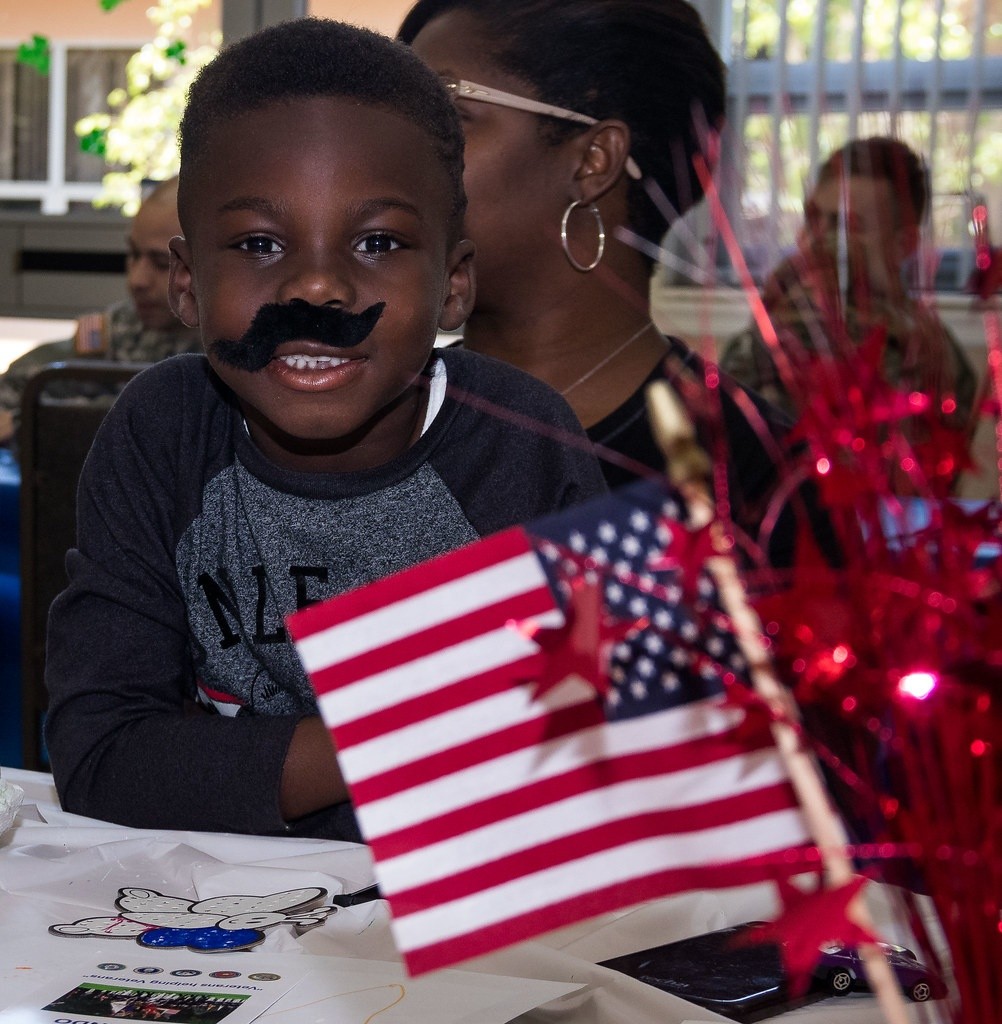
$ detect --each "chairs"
[19,363,174,772]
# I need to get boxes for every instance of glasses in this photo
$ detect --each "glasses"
[440,76,644,180]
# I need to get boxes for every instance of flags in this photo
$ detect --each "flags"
[283,470,829,977]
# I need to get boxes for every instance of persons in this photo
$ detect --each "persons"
[711,135,981,474]
[388,1,845,571]
[1,173,204,441]
[45,15,613,871]
